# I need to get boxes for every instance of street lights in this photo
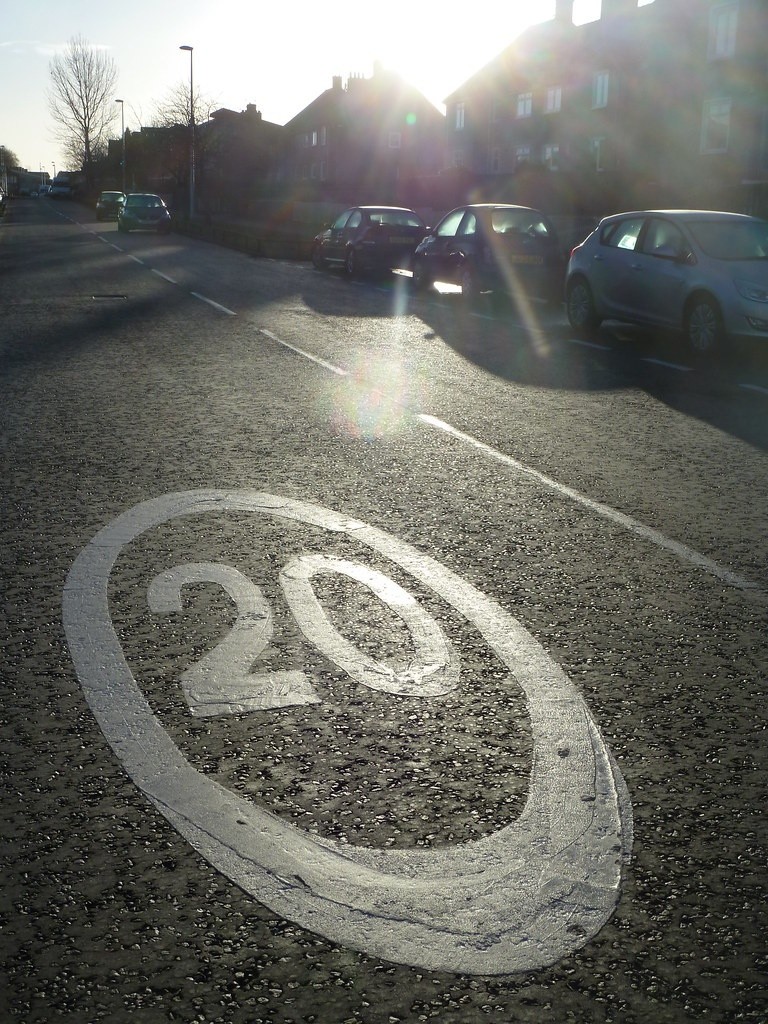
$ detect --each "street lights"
[178,44,194,237]
[115,99,125,191]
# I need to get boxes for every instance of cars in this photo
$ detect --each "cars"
[96,191,127,220]
[409,200,565,305]
[31,176,73,199]
[116,192,173,234]
[311,206,431,280]
[565,209,768,362]
